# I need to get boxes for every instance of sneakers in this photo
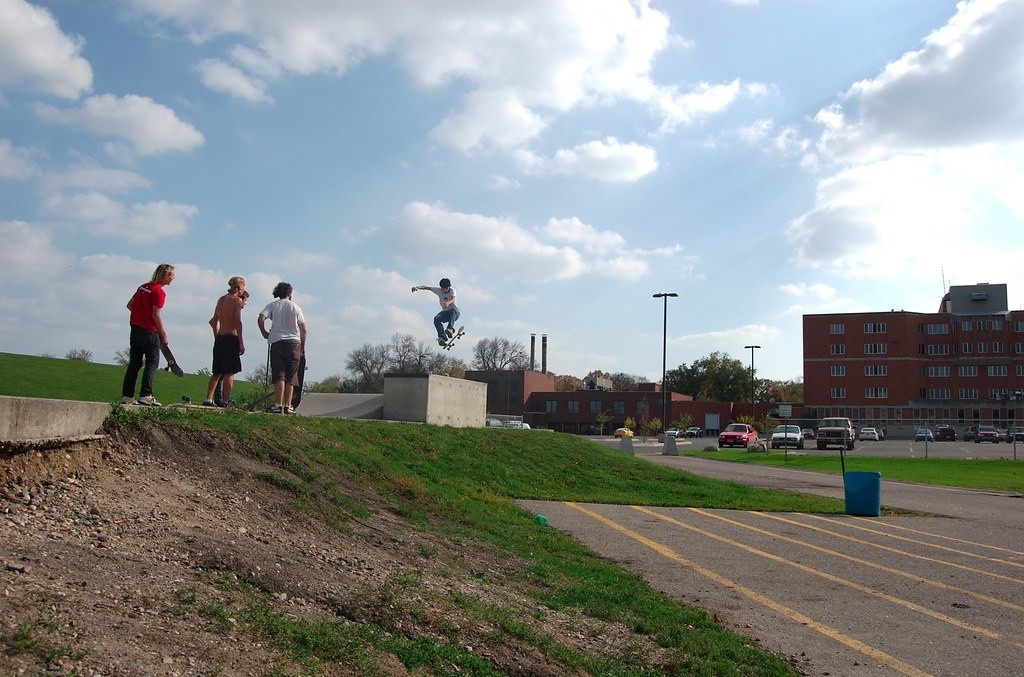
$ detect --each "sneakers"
[138,393,162,406]
[122,395,139,404]
[266,405,283,413]
[284,405,297,414]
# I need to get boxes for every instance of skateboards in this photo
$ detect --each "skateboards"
[167,395,226,410]
[290,355,308,408]
[214,378,224,403]
[443,326,465,351]
[159,335,183,377]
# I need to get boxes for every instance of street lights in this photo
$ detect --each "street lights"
[653,291,682,435]
[744,345,762,417]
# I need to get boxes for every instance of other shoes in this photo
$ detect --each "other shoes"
[438,338,447,346]
[204,399,219,407]
[445,329,453,338]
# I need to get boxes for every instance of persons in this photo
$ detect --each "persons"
[411,278,459,346]
[202,277,250,408]
[259,282,307,415]
[122,264,174,406]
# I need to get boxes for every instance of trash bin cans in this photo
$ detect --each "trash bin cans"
[843,470,882,517]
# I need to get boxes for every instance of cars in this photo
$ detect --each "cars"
[486,418,532,429]
[770,425,804,450]
[718,423,760,448]
[802,429,816,440]
[684,427,703,438]
[664,428,680,438]
[859,427,886,441]
[915,429,934,442]
[999,429,1008,442]
[614,427,634,439]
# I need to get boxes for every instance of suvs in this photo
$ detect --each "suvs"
[936,426,957,442]
[816,417,857,450]
[964,425,999,444]
[1006,426,1024,443]
